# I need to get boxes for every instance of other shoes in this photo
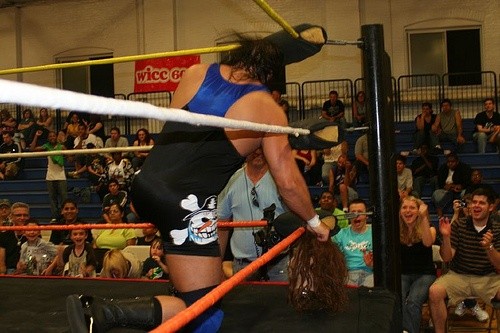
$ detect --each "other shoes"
[49,218,57,224]
[89,185,100,192]
[68,171,80,179]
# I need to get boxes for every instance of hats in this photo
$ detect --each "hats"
[0,199,10,206]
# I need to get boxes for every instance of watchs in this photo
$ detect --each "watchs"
[483,245,494,251]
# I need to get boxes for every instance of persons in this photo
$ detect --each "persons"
[0,90,500,333]
[66,24,330,333]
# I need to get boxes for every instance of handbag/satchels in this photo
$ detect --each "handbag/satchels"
[72,187,91,203]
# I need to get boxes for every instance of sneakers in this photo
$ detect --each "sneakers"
[471,305,489,321]
[455,302,470,318]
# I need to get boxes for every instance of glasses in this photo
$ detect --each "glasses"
[1,206,10,208]
[251,188,259,208]
[108,209,119,213]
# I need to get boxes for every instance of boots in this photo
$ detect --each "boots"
[66,294,162,333]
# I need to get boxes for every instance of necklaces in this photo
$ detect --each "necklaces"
[13,224,24,245]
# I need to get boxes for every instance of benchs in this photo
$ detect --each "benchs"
[0,117,500,305]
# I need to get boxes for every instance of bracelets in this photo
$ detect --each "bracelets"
[306,214,322,228]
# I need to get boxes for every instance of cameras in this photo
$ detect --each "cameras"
[460,200,468,208]
[255,203,283,247]
